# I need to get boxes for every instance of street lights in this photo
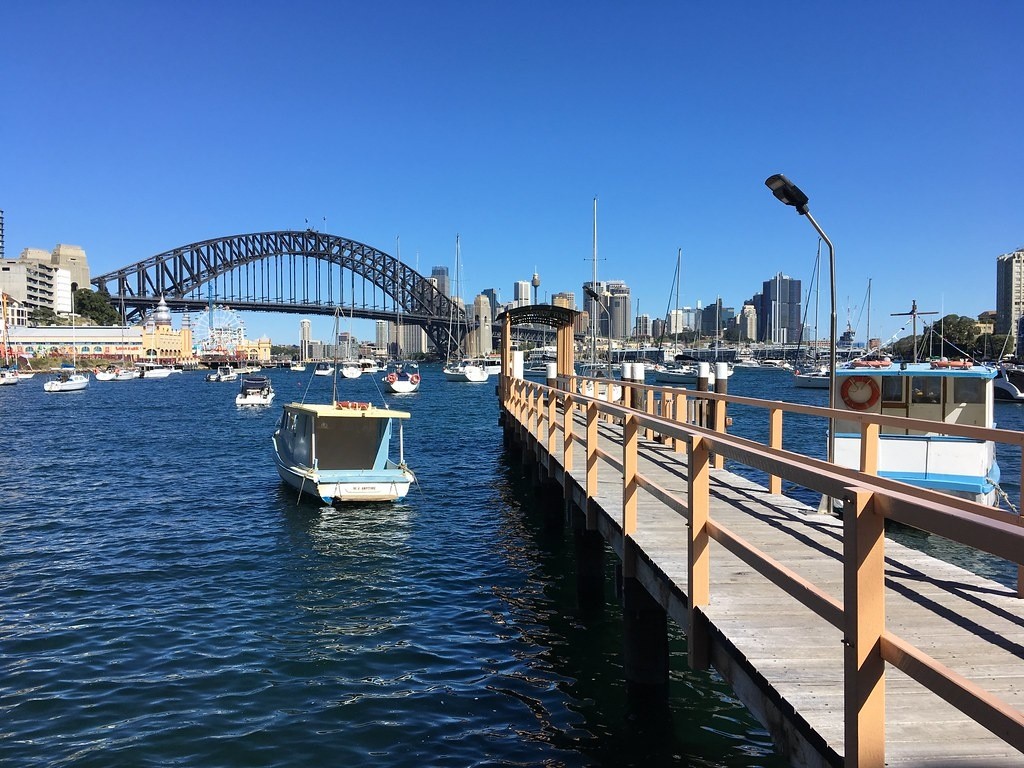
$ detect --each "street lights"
[765,173,833,516]
[581,284,613,380]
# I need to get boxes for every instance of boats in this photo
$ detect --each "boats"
[315,362,334,377]
[358,359,379,373]
[236,375,275,407]
[819,358,1001,528]
[729,331,760,368]
[289,363,305,371]
[0,372,19,385]
[204,365,237,381]
[479,357,502,375]
[234,360,261,373]
[271,303,416,505]
[374,360,388,372]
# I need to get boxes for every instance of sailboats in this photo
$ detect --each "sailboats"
[95,289,141,381]
[442,233,490,381]
[142,305,182,379]
[524,291,558,375]
[793,238,830,390]
[835,278,871,369]
[44,292,90,391]
[709,294,735,376]
[2,325,35,378]
[578,195,627,402]
[621,298,655,371]
[381,235,422,394]
[339,304,362,379]
[759,314,794,370]
[652,248,714,385]
[980,304,1024,403]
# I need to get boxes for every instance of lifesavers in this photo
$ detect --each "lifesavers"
[388,374,396,383]
[841,376,880,410]
[115,369,119,374]
[930,360,973,368]
[337,401,370,409]
[411,374,421,384]
[93,369,98,374]
[852,359,892,368]
